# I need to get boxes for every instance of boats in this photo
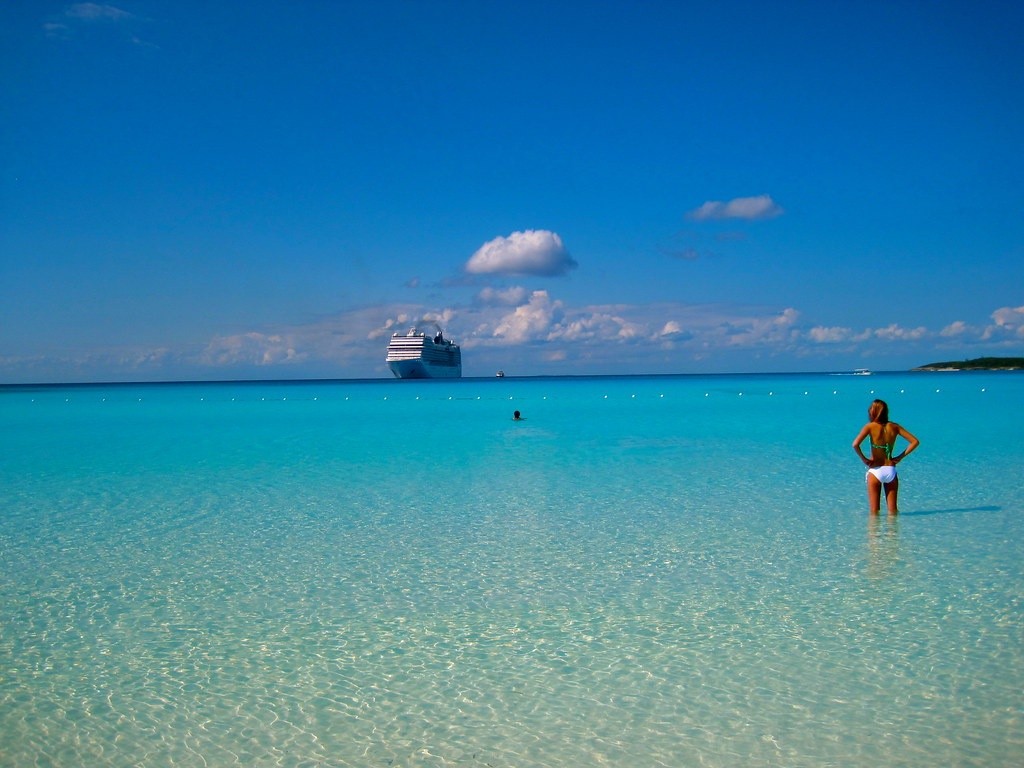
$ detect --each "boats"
[496,370,504,378]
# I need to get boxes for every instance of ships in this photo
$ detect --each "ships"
[385,323,463,379]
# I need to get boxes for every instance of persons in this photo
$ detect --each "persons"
[514,411,520,418]
[854,399,918,511]
